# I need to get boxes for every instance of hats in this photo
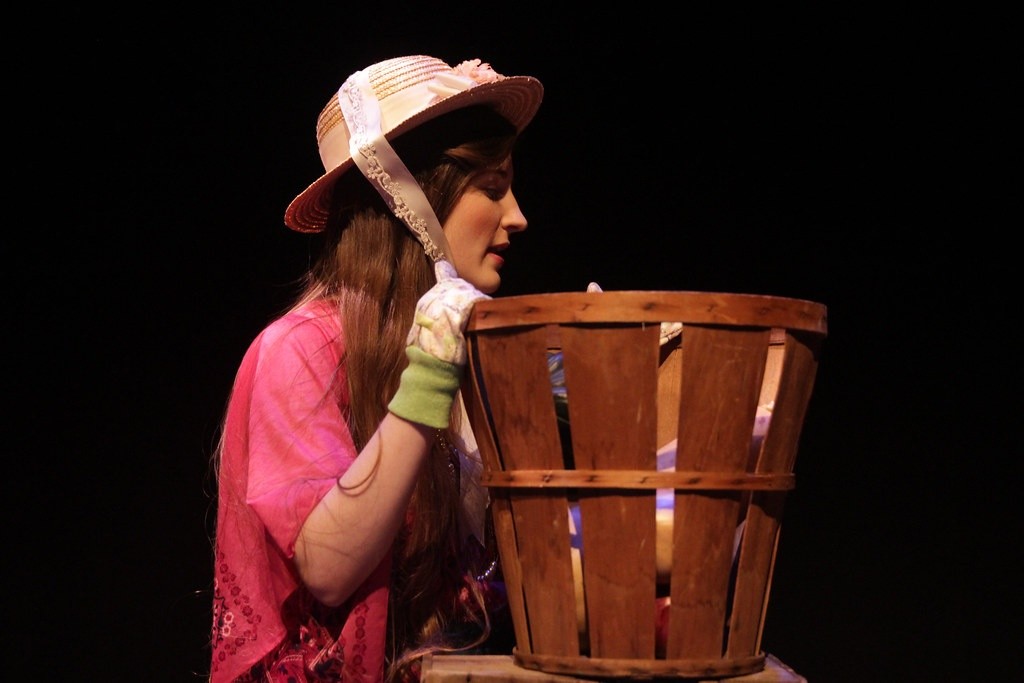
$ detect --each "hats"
[284,56,544,235]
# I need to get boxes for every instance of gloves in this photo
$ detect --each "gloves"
[386,260,492,430]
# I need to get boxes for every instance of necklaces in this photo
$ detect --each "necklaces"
[439,429,460,482]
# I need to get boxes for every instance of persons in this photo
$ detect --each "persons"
[209,52,604,683]
